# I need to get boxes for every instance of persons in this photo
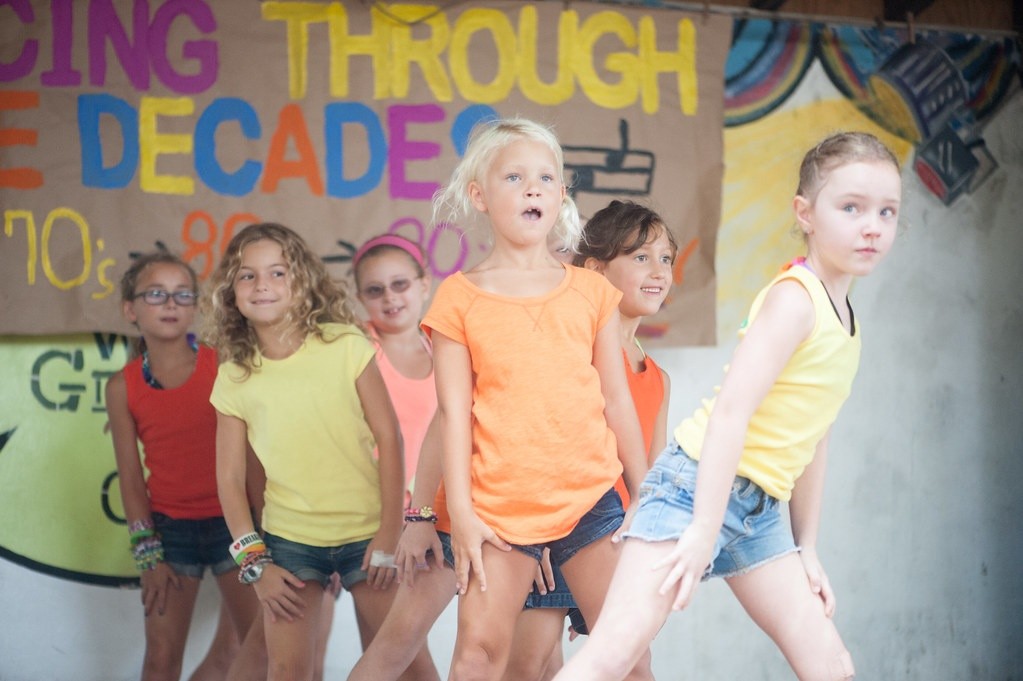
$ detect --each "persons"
[551,132,901,681]
[420,116,650,681]
[314,233,439,681]
[105,253,267,681]
[210,224,442,681]
[346,200,677,681]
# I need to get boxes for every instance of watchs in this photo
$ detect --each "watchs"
[241,565,263,584]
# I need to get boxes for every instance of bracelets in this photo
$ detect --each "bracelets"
[404,506,438,524]
[228,532,273,583]
[128,519,164,572]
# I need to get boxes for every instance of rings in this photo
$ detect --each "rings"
[416,563,427,567]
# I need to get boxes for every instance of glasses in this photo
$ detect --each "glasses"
[361,274,420,300]
[129,289,198,307]
